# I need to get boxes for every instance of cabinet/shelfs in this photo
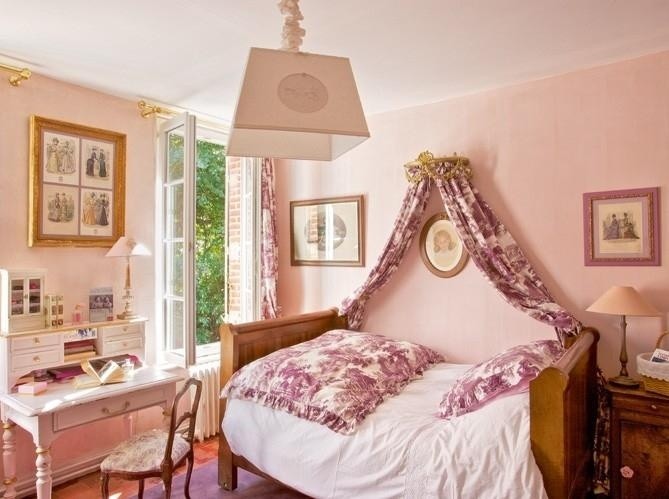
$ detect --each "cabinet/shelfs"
[1,314,149,393]
[601,367,669,499]
[1,267,48,332]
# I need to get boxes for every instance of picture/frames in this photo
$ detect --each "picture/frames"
[581,185,662,267]
[27,113,131,250]
[418,212,469,279]
[288,193,366,268]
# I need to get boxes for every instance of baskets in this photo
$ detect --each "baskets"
[635,331,669,397]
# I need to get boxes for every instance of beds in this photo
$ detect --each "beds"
[217,306,601,498]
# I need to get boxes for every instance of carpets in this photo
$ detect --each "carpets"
[127,453,308,499]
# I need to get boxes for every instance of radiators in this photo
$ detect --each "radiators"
[190,361,219,443]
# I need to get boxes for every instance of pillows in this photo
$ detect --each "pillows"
[434,337,569,421]
[220,325,447,438]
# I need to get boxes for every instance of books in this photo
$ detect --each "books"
[650,348,669,363]
[14,341,127,389]
[44,295,64,330]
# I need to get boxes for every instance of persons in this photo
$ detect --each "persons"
[604,213,640,240]
[47,137,109,226]
[91,296,112,309]
[434,230,458,267]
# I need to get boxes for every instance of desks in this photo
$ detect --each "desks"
[1,364,186,499]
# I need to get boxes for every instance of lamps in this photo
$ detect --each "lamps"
[584,285,664,388]
[227,1,371,164]
[104,235,154,320]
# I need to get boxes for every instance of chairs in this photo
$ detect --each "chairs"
[97,377,204,499]
